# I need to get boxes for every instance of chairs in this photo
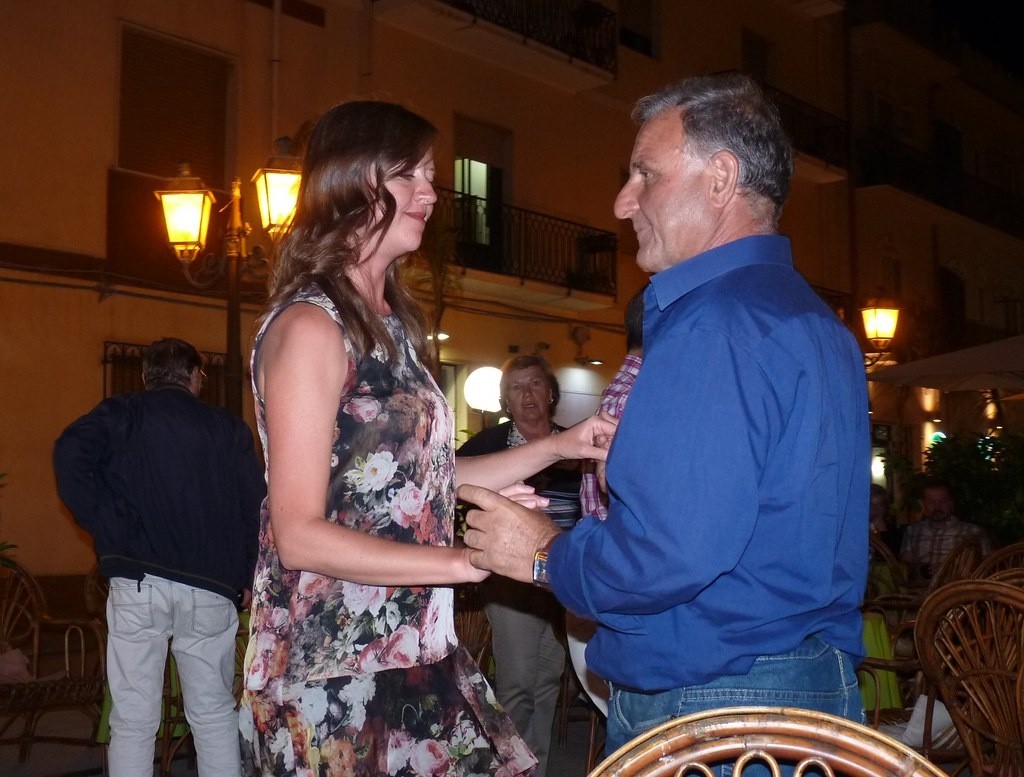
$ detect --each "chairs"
[0,558,250,777]
[456,532,1024,777]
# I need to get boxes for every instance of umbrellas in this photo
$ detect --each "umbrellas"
[867,333,1024,390]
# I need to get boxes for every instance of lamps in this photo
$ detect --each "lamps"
[575,356,604,366]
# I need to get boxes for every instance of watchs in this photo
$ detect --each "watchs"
[533,533,562,591]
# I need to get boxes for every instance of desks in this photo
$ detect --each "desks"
[858,612,900,711]
[98,609,250,745]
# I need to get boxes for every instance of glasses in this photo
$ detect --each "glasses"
[199,368,209,386]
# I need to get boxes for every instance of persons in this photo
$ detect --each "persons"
[870,483,993,576]
[457,353,580,777]
[54,337,265,777]
[457,73,873,777]
[565,284,649,719]
[239,99,620,777]
